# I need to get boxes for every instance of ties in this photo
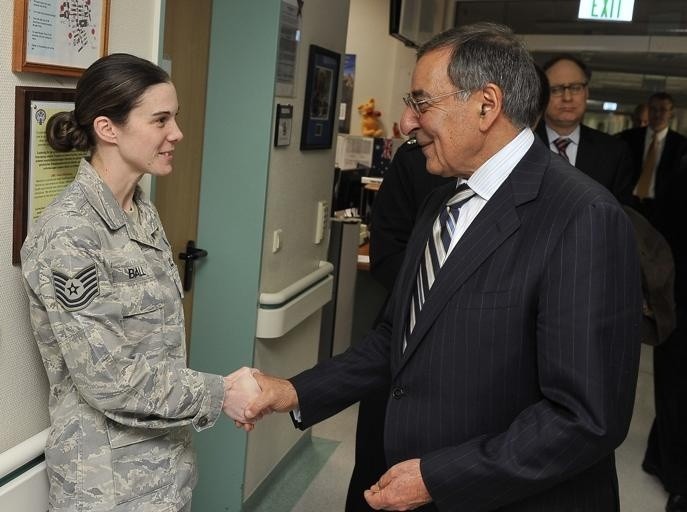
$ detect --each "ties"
[402,182,476,355]
[552,137,573,164]
[635,132,660,202]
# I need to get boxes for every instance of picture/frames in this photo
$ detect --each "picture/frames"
[299,45,341,150]
[12,86,92,266]
[12,0,111,76]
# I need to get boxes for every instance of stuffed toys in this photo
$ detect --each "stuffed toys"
[356,97,384,139]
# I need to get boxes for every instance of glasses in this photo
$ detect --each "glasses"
[403,84,476,117]
[550,80,589,95]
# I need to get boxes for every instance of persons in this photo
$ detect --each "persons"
[338,59,554,512]
[605,90,685,245]
[530,48,623,207]
[16,50,272,511]
[635,207,686,511]
[230,19,648,512]
[628,102,651,129]
